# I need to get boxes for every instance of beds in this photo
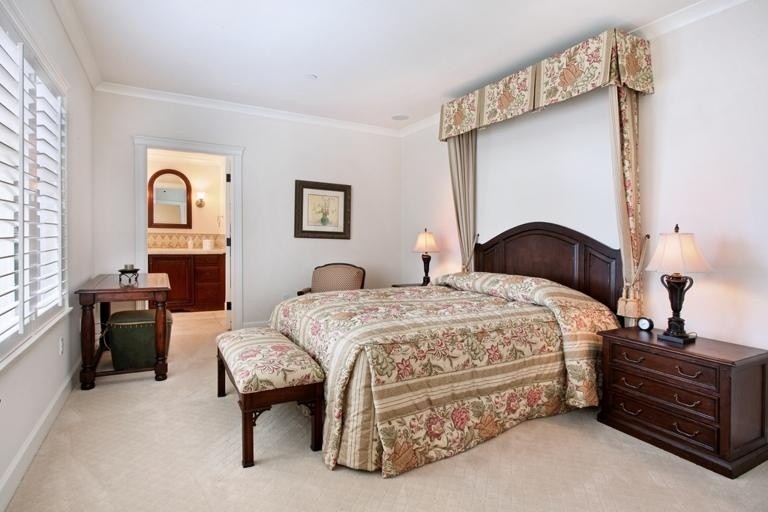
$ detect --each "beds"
[269,221,624,477]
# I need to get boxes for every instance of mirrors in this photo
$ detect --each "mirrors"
[147,169,192,229]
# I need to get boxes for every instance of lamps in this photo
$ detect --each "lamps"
[645,224,711,345]
[196,192,205,208]
[411,227,440,286]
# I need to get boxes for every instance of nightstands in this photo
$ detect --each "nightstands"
[596,326,767,479]
[392,283,437,288]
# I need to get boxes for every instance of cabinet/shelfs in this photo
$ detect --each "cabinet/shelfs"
[149,254,224,311]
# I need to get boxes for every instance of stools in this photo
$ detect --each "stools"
[107,310,173,369]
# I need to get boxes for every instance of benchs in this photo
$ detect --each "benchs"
[215,326,326,468]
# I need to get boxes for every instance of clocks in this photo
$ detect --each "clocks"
[636,317,653,331]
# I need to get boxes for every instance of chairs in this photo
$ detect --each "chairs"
[297,263,366,296]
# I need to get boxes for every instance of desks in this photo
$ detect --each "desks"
[73,273,171,390]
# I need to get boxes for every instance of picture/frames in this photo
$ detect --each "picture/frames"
[294,179,351,239]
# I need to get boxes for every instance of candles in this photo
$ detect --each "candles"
[125,265,134,270]
